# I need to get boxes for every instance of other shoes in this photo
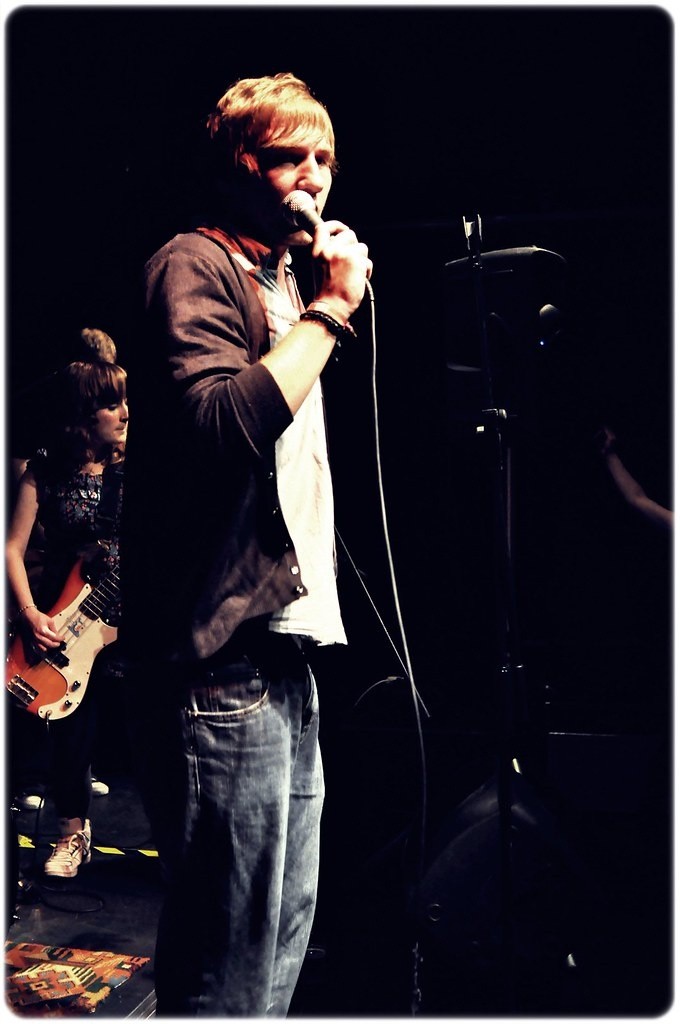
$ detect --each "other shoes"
[20,793,46,810]
[91,775,110,796]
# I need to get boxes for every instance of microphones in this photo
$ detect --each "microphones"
[282,190,372,294]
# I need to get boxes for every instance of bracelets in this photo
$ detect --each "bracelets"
[300,300,357,346]
[17,604,37,616]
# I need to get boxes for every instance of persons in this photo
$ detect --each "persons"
[7,329,129,879]
[120,74,372,1018]
[524,388,669,576]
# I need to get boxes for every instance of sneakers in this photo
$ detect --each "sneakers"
[44,817,91,880]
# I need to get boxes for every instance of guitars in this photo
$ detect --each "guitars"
[5,555,121,724]
